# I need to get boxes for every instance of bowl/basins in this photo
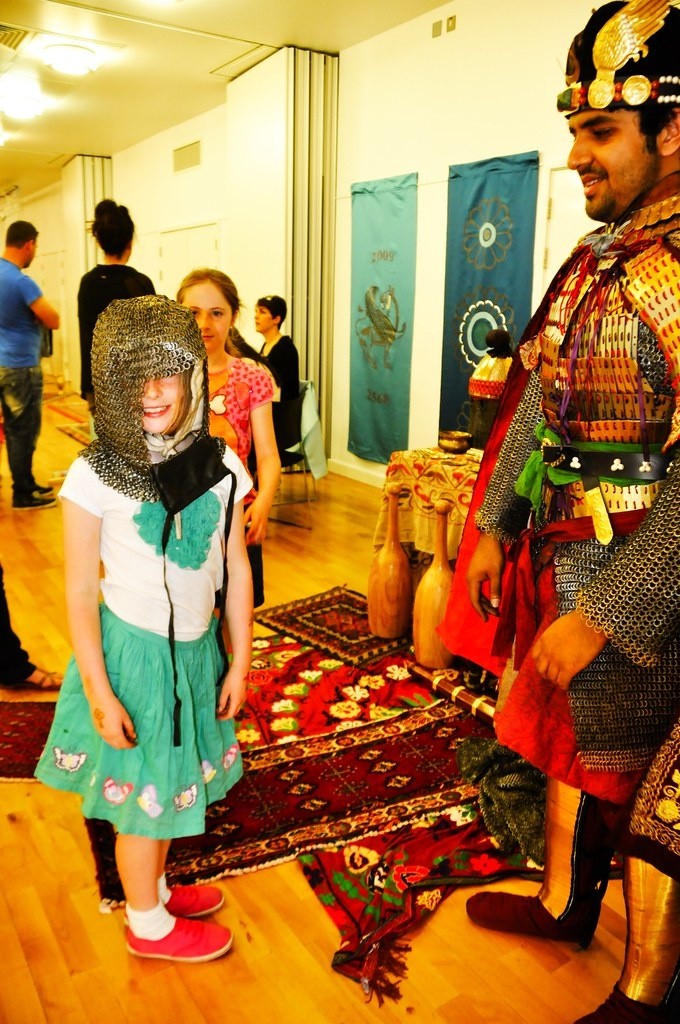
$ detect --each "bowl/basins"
[438,431,472,453]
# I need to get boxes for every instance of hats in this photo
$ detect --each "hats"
[557,1,680,119]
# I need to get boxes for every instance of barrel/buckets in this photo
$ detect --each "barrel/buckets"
[470,394,515,448]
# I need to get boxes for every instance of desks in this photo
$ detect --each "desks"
[375,449,484,563]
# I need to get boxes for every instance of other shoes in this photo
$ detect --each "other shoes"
[566,989,664,1024]
[11,495,57,512]
[31,485,53,498]
[466,890,576,942]
[122,884,224,927]
[125,918,232,962]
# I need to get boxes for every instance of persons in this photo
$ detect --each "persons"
[0,562,65,690]
[0,220,59,510]
[253,294,301,453]
[439,0,680,1024]
[78,200,156,439]
[176,269,283,719]
[33,293,257,964]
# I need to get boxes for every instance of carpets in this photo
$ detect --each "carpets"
[219,636,624,1003]
[57,422,92,448]
[1,698,55,777]
[86,701,520,911]
[256,586,412,665]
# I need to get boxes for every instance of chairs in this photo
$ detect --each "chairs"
[252,379,321,530]
